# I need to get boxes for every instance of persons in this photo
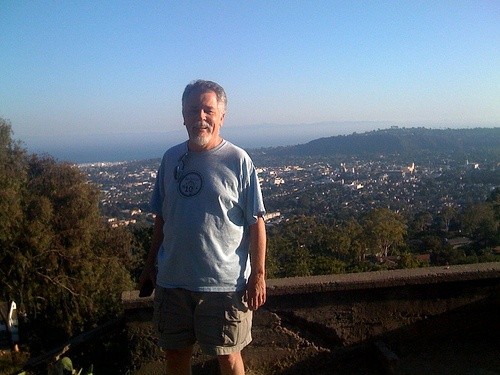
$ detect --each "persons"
[138,80,267,375]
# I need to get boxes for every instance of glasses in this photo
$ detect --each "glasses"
[174,151,190,181]
